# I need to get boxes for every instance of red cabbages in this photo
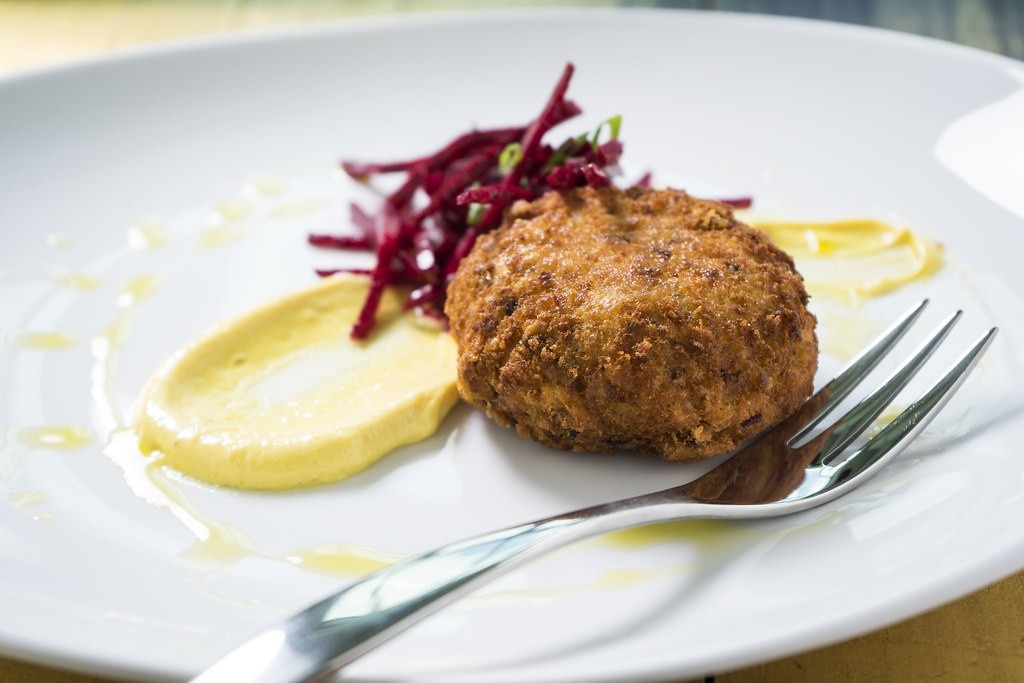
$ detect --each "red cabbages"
[305,60,754,341]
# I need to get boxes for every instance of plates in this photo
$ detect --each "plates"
[0,11,1024,682]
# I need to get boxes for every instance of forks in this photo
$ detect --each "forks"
[184,298,1001,683]
